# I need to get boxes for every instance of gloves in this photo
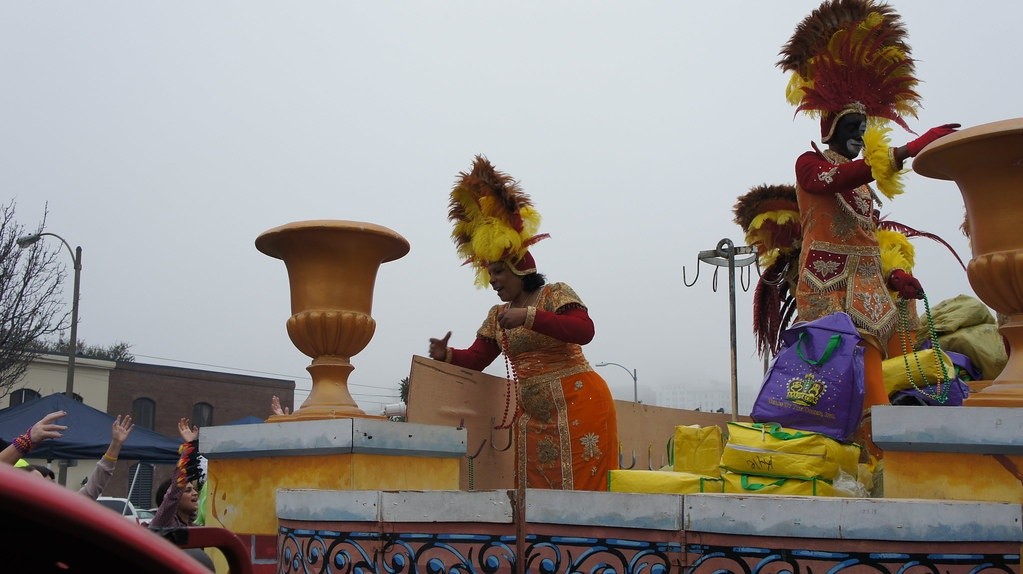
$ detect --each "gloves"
[887,269,924,300]
[907,123,961,157]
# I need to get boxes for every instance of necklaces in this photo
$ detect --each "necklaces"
[495,329,520,429]
[898,292,949,404]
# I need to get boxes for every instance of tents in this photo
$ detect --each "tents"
[0,392,184,517]
[178,415,265,443]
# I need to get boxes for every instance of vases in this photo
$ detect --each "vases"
[912,119,1023,407]
[255,222,411,421]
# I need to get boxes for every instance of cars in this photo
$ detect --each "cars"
[136,509,155,524]
[97,496,139,525]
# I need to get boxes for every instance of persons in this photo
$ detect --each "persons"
[797,101,962,462]
[430,247,620,491]
[149,417,199,528]
[884,299,919,357]
[33,414,134,501]
[0,410,67,480]
[271,396,288,416]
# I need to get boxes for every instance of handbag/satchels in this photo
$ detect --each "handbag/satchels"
[882,339,983,407]
[608,421,876,497]
[750,311,865,442]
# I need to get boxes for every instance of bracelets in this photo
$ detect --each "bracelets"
[13,425,33,453]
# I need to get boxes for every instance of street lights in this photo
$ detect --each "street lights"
[16,232,82,487]
[596,362,638,403]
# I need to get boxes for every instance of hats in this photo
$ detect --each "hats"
[774,0,925,144]
[447,154,550,289]
[733,184,801,266]
